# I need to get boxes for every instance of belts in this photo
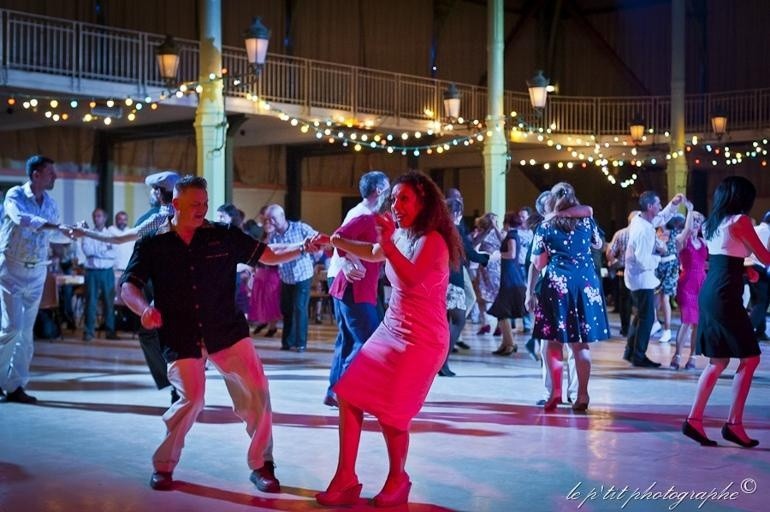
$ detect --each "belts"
[6,255,52,268]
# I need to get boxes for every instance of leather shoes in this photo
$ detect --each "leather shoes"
[721,422,759,448]
[682,416,716,446]
[624,321,697,369]
[83,334,93,341]
[106,334,120,340]
[7,386,36,402]
[265,328,277,337]
[457,342,470,349]
[250,460,279,493]
[0,387,6,402]
[254,323,267,333]
[150,469,173,490]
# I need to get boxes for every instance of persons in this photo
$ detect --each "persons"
[681,173,770,448]
[303,169,468,507]
[121,174,319,494]
[0,150,769,415]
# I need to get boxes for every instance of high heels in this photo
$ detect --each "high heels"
[571,396,591,410]
[373,474,411,507]
[478,325,490,333]
[544,397,561,411]
[493,344,517,355]
[494,326,502,335]
[316,480,363,506]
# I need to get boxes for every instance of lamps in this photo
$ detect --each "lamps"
[505,69,551,120]
[155,15,272,94]
[686,104,728,142]
[443,83,484,131]
[628,113,671,148]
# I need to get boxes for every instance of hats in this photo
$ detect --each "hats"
[145,171,180,191]
